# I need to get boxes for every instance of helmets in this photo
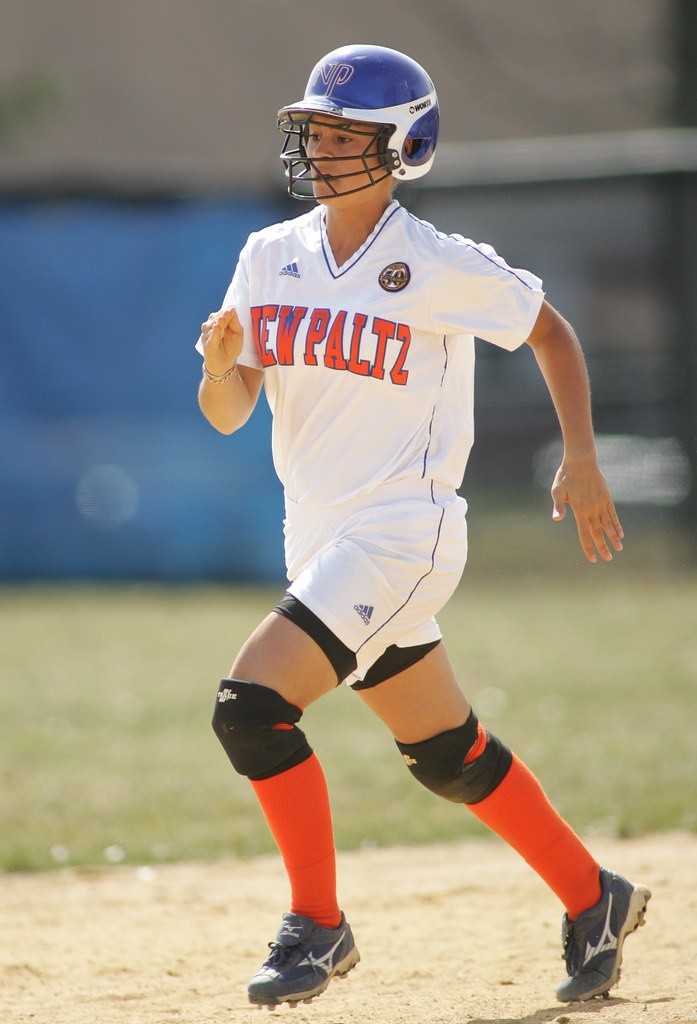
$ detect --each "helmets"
[278,44,440,181]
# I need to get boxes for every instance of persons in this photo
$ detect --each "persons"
[196,44,651,1006]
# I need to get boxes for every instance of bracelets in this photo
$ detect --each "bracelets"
[200,363,236,383]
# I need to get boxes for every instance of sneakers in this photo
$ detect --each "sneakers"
[247,910,363,1010]
[556,866,652,1003]
